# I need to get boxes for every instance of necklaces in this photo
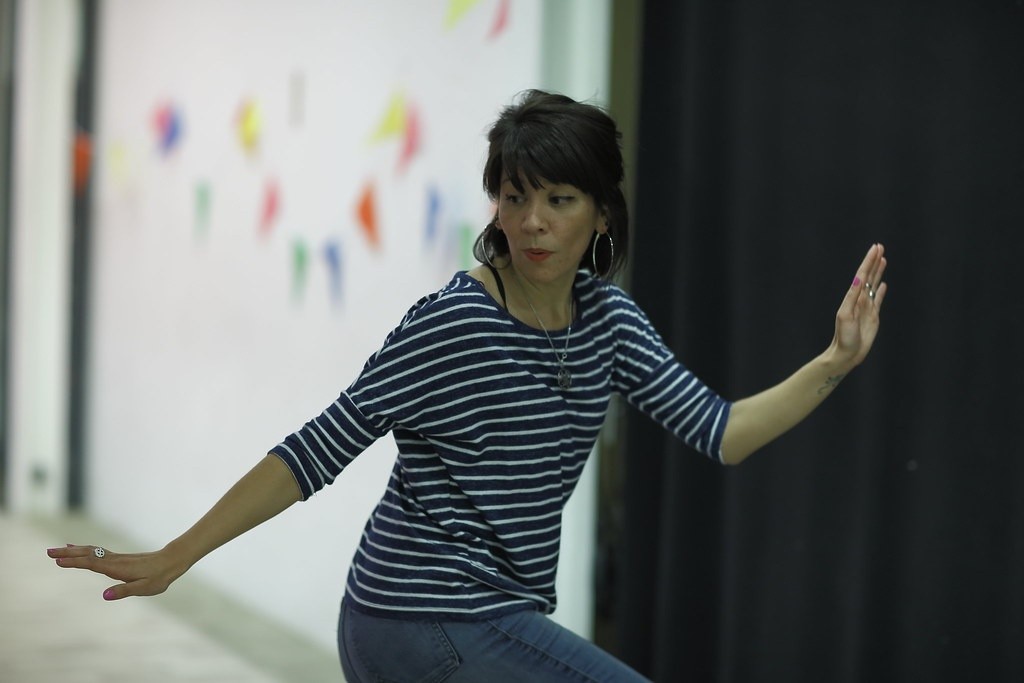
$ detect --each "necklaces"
[522,291,572,391]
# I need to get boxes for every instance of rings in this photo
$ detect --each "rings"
[866,283,872,288]
[95,548,105,558]
[869,291,875,298]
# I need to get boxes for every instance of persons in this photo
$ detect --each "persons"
[47,89,886,683]
